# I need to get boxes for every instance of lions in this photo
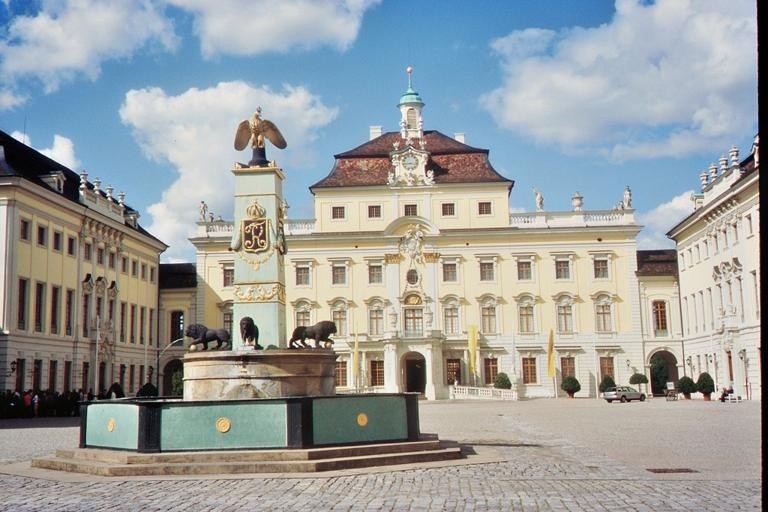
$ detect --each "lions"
[239,316,260,345]
[288,320,339,349]
[183,321,231,354]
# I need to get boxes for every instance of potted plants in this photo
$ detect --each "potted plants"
[561,373,580,398]
[696,371,716,401]
[676,375,698,400]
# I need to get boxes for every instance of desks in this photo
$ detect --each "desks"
[662,389,679,402]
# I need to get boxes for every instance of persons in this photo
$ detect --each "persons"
[0,386,104,418]
[719,386,734,402]
[198,200,208,221]
[623,185,633,206]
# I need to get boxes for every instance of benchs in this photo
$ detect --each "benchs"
[723,391,739,403]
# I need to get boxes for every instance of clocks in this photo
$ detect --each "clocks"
[401,153,419,170]
